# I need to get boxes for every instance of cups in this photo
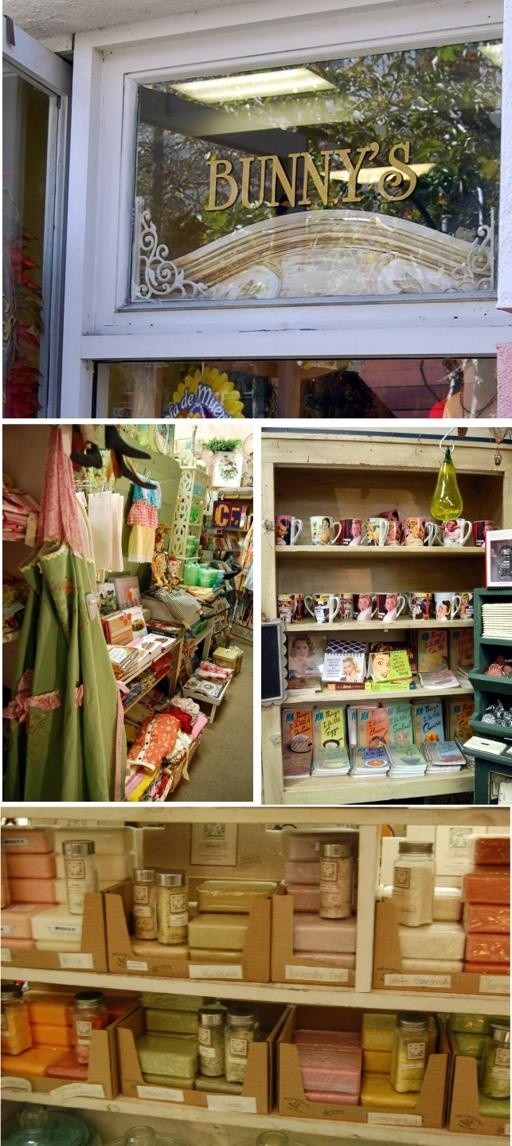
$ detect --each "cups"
[275,508,501,547]
[277,591,474,625]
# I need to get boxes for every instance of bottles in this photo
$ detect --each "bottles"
[429,446,463,521]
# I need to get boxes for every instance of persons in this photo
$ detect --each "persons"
[378,594,397,622]
[436,600,451,619]
[275,518,293,545]
[315,517,335,547]
[347,520,364,547]
[354,594,372,621]
[420,594,432,618]
[443,520,461,542]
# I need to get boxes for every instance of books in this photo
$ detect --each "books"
[277,638,477,782]
[95,574,183,690]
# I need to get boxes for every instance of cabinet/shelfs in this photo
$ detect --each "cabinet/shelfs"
[1,424,510,1146]
